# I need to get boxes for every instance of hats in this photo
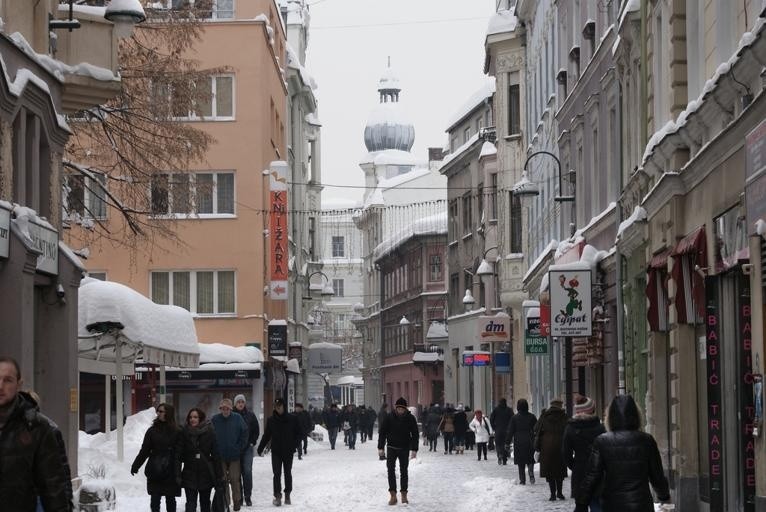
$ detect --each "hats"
[575,395,596,415]
[218,394,246,410]
[395,397,407,409]
[550,398,563,408]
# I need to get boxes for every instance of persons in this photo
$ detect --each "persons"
[231,394,259,506]
[180,407,225,512]
[378,397,419,506]
[560,393,608,511]
[259,398,302,506]
[532,400,570,502]
[572,394,672,511]
[0,357,73,512]
[130,402,185,512]
[209,399,249,512]
[506,398,538,485]
[290,403,377,461]
[469,410,493,461]
[490,397,514,465]
[420,401,475,455]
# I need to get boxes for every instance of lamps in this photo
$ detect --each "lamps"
[461,282,486,307]
[512,151,575,206]
[398,307,422,328]
[475,245,499,277]
[45,0,147,34]
[301,270,334,300]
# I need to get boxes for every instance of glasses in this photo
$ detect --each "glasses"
[157,411,166,413]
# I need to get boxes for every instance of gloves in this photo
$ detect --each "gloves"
[379,451,385,459]
[411,451,416,459]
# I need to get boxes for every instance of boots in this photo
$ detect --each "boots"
[401,488,408,504]
[389,488,397,505]
[456,445,463,454]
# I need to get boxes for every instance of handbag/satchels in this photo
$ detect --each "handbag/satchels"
[533,432,541,463]
[212,483,230,511]
[144,450,171,479]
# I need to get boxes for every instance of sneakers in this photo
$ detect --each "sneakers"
[234,496,252,510]
[273,491,282,506]
[285,492,290,504]
